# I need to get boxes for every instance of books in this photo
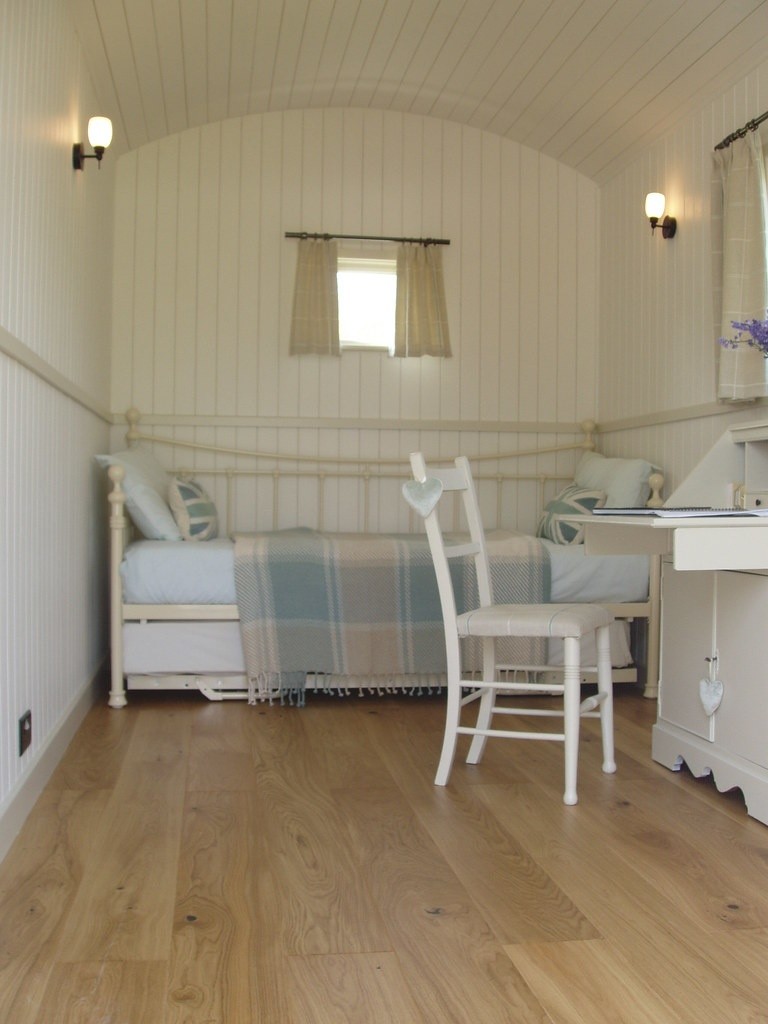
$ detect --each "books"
[592,506,743,516]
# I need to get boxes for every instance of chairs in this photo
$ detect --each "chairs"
[409,451,618,805]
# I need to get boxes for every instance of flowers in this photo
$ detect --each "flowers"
[719,318,768,359]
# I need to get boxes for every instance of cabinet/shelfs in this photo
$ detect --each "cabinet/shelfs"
[554,419,768,827]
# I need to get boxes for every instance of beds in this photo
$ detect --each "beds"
[106,406,665,710]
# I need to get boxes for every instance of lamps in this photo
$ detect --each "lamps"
[72,117,112,170]
[645,193,677,238]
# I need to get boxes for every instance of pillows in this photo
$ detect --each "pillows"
[168,477,219,541]
[93,439,185,541]
[574,450,662,508]
[536,481,608,545]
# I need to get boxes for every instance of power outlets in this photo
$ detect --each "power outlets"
[19,710,32,756]
